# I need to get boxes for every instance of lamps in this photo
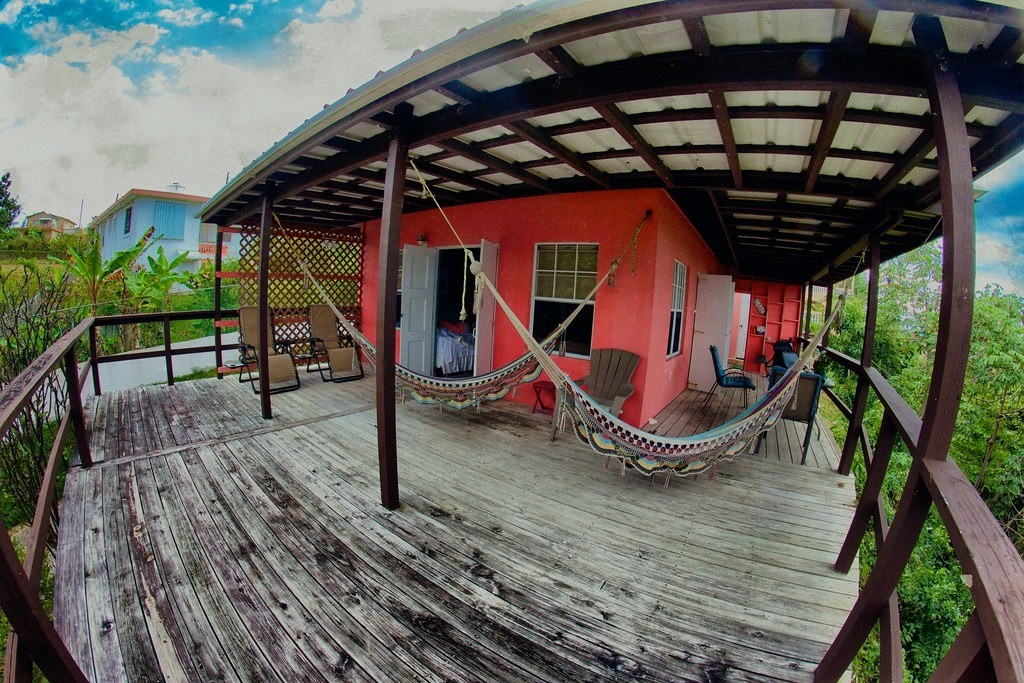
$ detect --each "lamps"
[415,233,428,247]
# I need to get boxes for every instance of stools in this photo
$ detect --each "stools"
[531,380,556,413]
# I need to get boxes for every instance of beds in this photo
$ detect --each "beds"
[434,333,474,374]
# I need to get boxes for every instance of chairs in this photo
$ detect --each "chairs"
[764,340,793,367]
[238,305,301,395]
[754,365,825,465]
[701,345,756,410]
[549,348,642,441]
[307,304,364,382]
[782,351,799,368]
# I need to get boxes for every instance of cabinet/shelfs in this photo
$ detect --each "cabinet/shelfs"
[735,278,803,374]
[687,271,752,395]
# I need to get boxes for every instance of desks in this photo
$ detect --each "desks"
[801,368,836,441]
